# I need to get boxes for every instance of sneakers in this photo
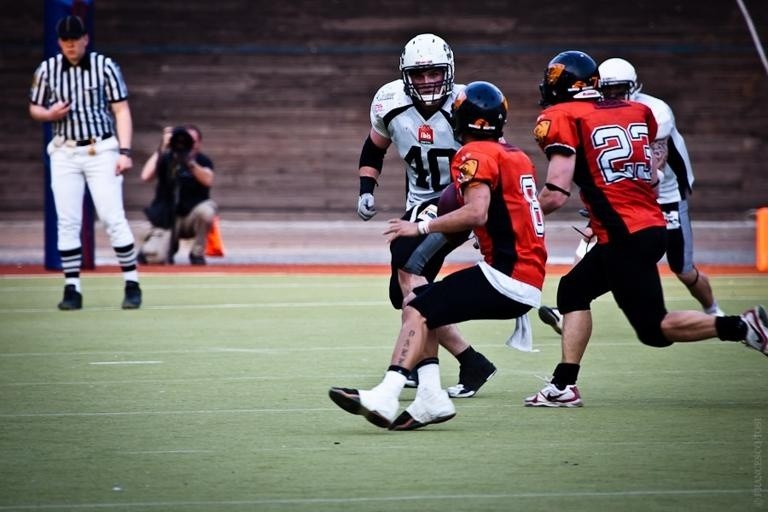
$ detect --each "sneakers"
[330,386,400,426]
[388,391,455,434]
[526,384,583,409]
[189,252,205,265]
[540,306,564,333]
[738,308,768,355]
[59,284,82,310]
[448,355,496,398]
[122,283,142,309]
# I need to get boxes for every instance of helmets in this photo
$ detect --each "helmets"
[453,81,508,140]
[540,51,641,103]
[400,34,455,109]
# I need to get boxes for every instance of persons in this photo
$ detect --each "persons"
[326,80,548,432]
[137,123,218,266]
[354,32,498,401]
[27,14,143,311]
[537,56,727,336]
[524,49,768,409]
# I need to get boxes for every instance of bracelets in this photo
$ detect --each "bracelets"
[417,220,431,235]
[120,149,133,157]
[358,175,377,195]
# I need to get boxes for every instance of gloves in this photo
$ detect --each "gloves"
[357,176,377,221]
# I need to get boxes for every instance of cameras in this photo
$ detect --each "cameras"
[169,127,194,154]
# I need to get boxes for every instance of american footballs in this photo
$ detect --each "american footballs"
[437,183,471,244]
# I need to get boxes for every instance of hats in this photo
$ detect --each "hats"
[57,17,85,41]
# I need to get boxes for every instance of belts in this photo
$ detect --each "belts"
[77,132,112,146]
